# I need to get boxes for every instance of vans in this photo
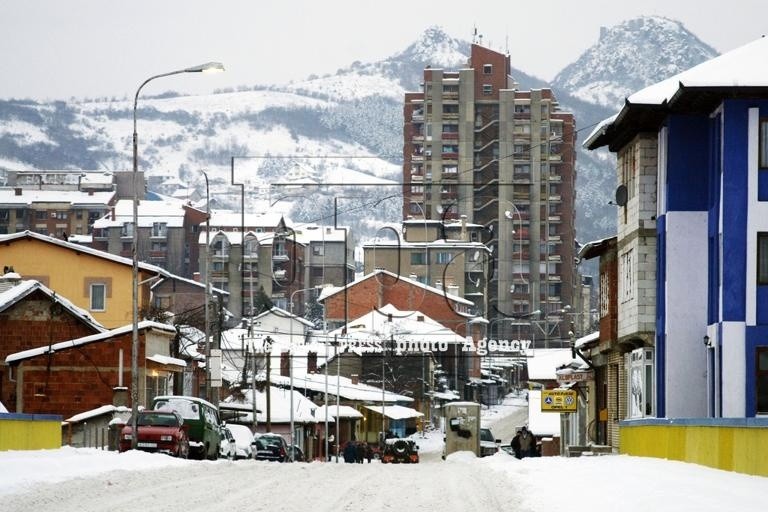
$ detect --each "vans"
[149,395,226,461]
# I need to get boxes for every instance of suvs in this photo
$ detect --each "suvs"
[381,437,420,463]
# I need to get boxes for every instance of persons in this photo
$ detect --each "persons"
[325,427,400,465]
[526,431,537,457]
[518,426,532,459]
[510,430,522,459]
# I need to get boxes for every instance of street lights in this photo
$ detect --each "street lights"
[131,59,226,452]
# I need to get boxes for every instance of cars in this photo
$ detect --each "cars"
[441,426,503,457]
[116,408,191,459]
[217,423,306,463]
